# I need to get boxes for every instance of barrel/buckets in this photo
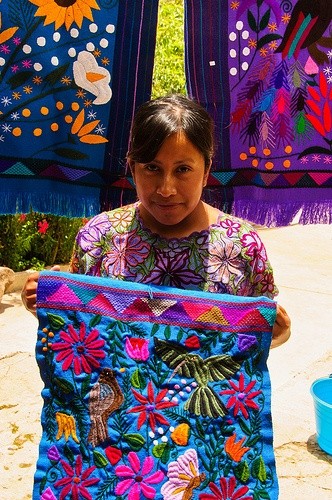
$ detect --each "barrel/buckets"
[311,373,332,457]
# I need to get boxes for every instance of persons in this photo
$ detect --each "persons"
[21,95,292,349]
